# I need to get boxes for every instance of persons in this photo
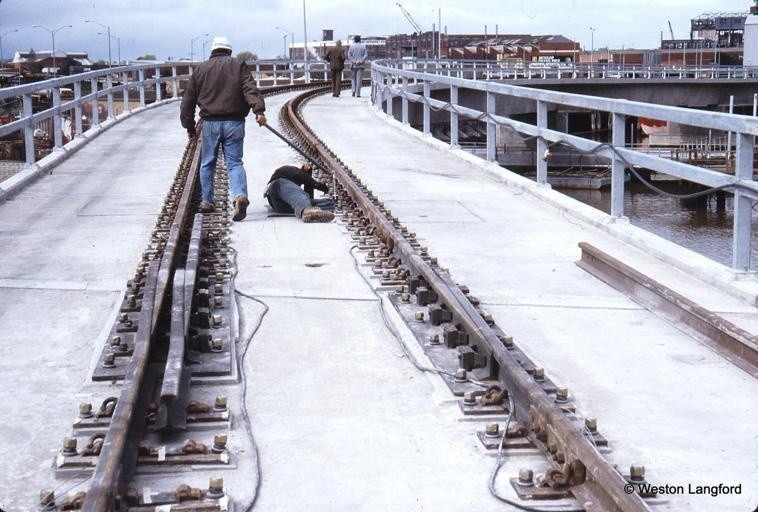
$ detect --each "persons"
[180,36,335,223]
[325,36,368,97]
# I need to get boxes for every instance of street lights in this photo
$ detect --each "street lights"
[0,30,17,79]
[32,25,72,77]
[191,33,208,61]
[85,19,121,67]
[275,27,294,61]
[590,28,595,51]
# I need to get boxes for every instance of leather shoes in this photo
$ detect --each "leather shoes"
[352,92,361,97]
[332,94,339,97]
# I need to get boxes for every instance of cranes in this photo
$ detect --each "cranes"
[396,2,426,34]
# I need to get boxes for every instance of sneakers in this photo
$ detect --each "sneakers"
[201,201,215,212]
[302,205,335,223]
[232,196,249,221]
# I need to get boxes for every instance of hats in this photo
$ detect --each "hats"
[210,36,232,52]
[334,40,344,44]
